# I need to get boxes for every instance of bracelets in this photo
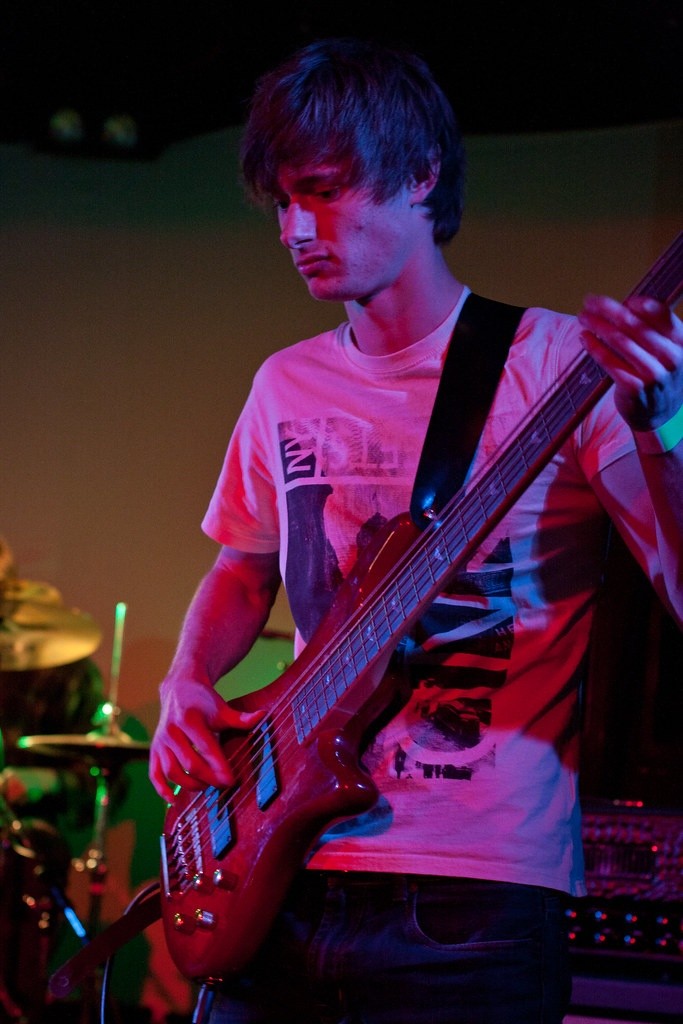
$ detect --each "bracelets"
[633,404,683,456]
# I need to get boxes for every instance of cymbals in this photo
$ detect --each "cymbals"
[18,730,148,760]
[0,597,101,670]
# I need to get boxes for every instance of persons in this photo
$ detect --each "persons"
[148,38,683,1024]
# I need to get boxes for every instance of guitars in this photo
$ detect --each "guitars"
[158,234,683,983]
